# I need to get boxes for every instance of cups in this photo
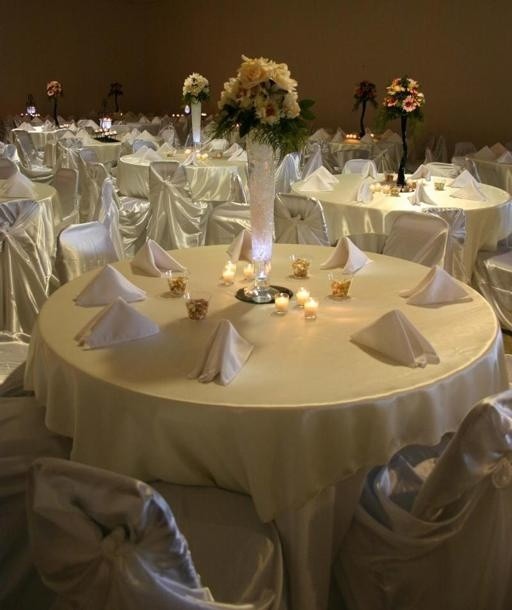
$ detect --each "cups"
[290,254,312,278]
[166,269,189,296]
[390,183,402,198]
[329,272,352,300]
[252,256,272,303]
[185,292,211,320]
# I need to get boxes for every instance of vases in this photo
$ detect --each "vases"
[190,99,202,144]
[243,129,278,304]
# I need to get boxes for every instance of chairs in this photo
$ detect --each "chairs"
[331,391,512,610]
[58,222,119,282]
[50,169,78,228]
[0,143,20,173]
[273,193,329,246]
[206,201,249,245]
[14,128,55,186]
[148,161,204,249]
[425,162,456,179]
[79,149,96,172]
[273,152,299,191]
[302,151,321,178]
[0,396,74,551]
[0,341,31,393]
[380,213,449,271]
[59,177,122,277]
[24,455,287,610]
[0,198,62,336]
[133,139,157,154]
[344,159,376,175]
[426,208,466,281]
[478,251,512,330]
[93,163,150,256]
[453,158,479,181]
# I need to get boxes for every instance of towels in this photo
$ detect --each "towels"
[399,265,470,305]
[134,146,149,157]
[226,229,253,260]
[130,127,141,136]
[498,151,511,164]
[73,266,148,306]
[131,238,185,278]
[188,320,253,384]
[315,166,338,184]
[223,144,241,156]
[448,170,479,188]
[299,173,332,192]
[408,182,436,206]
[5,180,36,199]
[140,129,151,138]
[319,237,369,275]
[490,144,505,156]
[409,164,432,182]
[81,135,95,146]
[362,160,376,179]
[386,133,403,144]
[449,180,487,202]
[19,122,35,131]
[310,127,330,139]
[157,144,175,154]
[350,310,439,366]
[139,116,149,122]
[75,297,159,351]
[380,129,394,139]
[332,132,345,141]
[142,149,163,161]
[350,180,372,202]
[6,173,32,185]
[359,134,374,145]
[474,146,493,160]
[229,149,250,163]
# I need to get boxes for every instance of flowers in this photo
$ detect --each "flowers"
[352,80,380,136]
[212,55,315,159]
[45,80,63,127]
[109,83,123,111]
[377,75,427,185]
[180,73,210,104]
